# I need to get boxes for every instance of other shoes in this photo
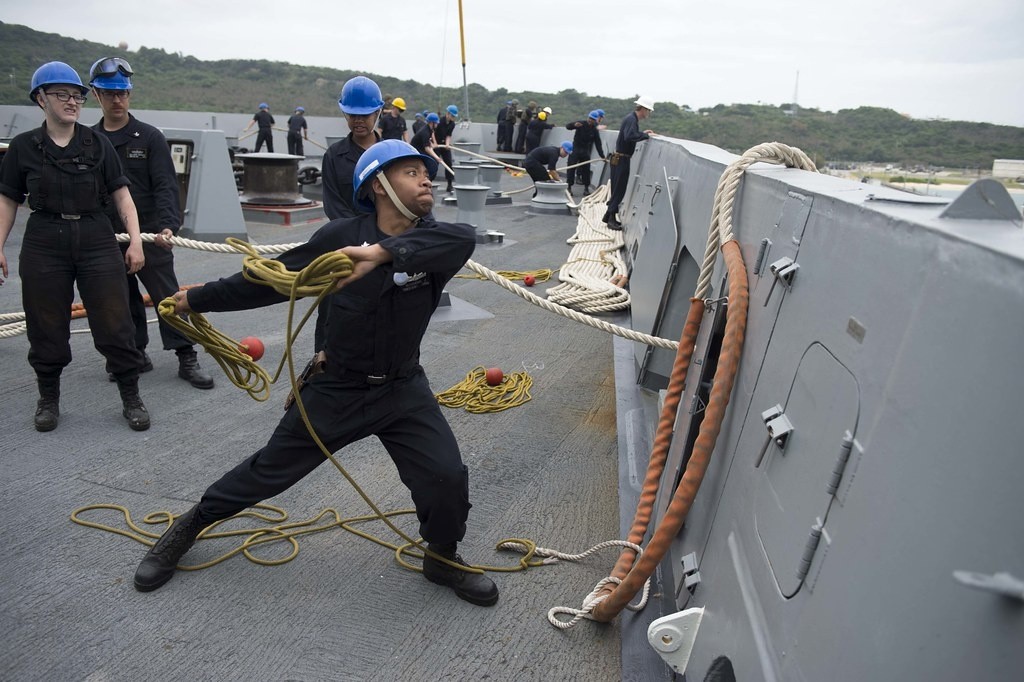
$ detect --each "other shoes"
[583,189,591,195]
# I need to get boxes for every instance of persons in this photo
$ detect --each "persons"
[0,61,151,433]
[377,98,409,143]
[566,109,605,196]
[321,76,435,224]
[431,105,459,192]
[411,110,440,154]
[496,99,556,154]
[523,141,573,197]
[286,106,307,155]
[243,103,274,153]
[87,57,214,389]
[134,140,499,607]
[602,95,658,230]
[379,94,393,118]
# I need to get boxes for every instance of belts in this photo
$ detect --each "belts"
[37,210,96,220]
[323,359,420,386]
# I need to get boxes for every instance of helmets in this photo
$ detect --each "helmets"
[415,105,458,124]
[562,140,573,155]
[353,139,437,214]
[339,76,385,114]
[634,96,655,111]
[259,103,270,108]
[507,98,551,120]
[596,108,605,118]
[89,57,132,90]
[28,61,90,103]
[296,107,303,112]
[391,98,406,111]
[588,110,599,120]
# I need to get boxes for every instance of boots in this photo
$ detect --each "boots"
[35,379,60,431]
[114,376,150,431]
[135,504,202,592]
[609,215,623,230]
[603,212,621,225]
[423,540,498,605]
[138,347,155,374]
[177,350,215,389]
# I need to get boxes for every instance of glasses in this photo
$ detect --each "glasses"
[90,58,133,83]
[38,92,89,103]
[97,90,130,98]
